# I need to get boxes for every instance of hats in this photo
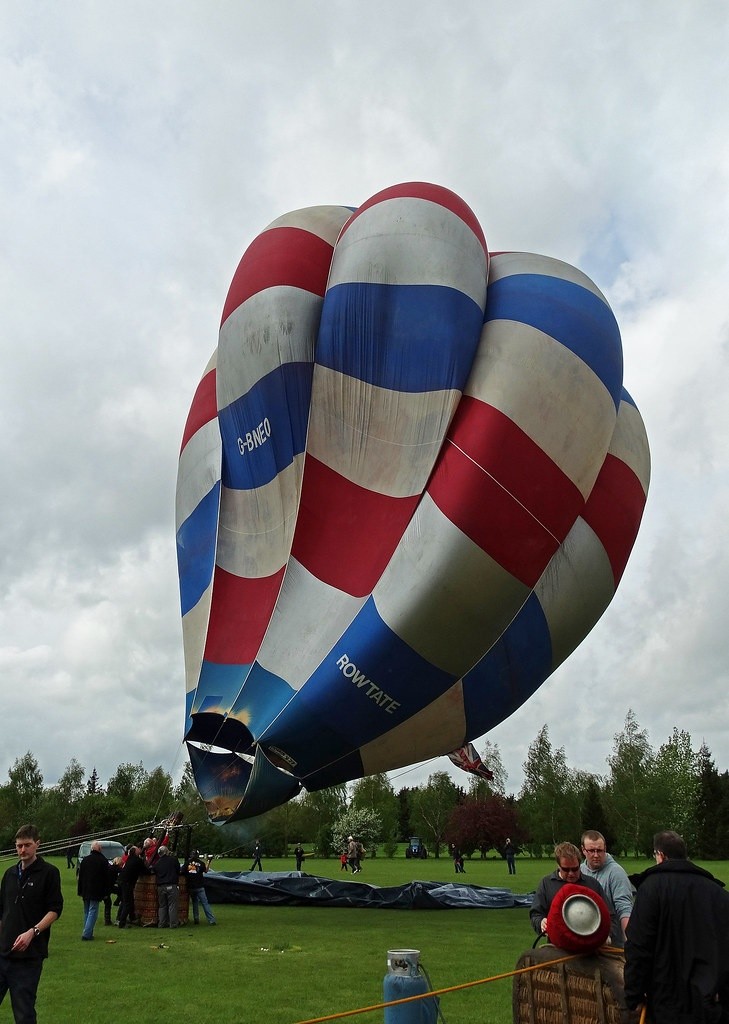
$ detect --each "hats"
[158,846,170,857]
[347,836,353,840]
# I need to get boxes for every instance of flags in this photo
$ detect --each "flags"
[447,743,494,781]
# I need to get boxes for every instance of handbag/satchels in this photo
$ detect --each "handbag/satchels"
[299,856,305,861]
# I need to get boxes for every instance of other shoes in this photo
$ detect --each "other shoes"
[351,870,357,874]
[114,920,120,925]
[359,870,360,872]
[210,921,216,926]
[249,868,253,871]
[195,921,199,925]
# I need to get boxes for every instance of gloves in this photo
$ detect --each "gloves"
[207,857,212,863]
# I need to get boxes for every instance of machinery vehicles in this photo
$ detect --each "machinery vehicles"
[406,837,427,859]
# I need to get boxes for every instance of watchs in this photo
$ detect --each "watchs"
[34,926,40,936]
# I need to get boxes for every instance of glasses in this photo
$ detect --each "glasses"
[557,862,580,872]
[652,850,658,859]
[583,846,605,854]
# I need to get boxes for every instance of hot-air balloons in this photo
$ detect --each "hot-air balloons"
[114,178,652,926]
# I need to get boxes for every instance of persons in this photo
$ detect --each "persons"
[0,824,63,1024]
[579,830,634,948]
[249,839,262,871]
[294,843,304,871]
[503,837,516,875]
[66,844,75,869]
[529,842,626,948]
[102,824,216,929]
[452,843,466,873]
[623,831,729,1024]
[77,841,110,941]
[340,836,363,874]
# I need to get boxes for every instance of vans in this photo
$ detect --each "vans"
[77,840,126,879]
